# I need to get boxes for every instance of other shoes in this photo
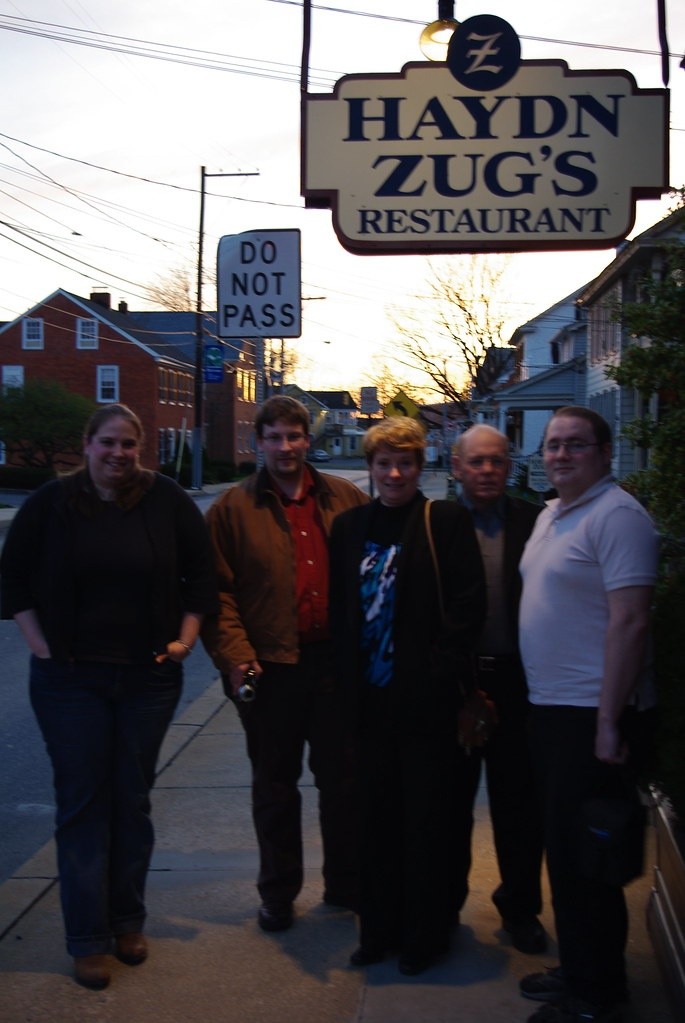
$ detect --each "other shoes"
[258,897,296,933]
[347,943,386,968]
[324,889,363,914]
[518,972,572,1001]
[523,1003,614,1023]
[395,931,449,973]
[502,914,549,956]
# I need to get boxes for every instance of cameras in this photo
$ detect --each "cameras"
[237,670,256,701]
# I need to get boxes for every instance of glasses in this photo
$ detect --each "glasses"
[262,431,306,442]
[542,441,603,453]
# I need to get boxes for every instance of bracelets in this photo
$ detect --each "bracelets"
[177,639,192,656]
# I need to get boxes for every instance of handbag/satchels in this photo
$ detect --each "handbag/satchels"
[457,680,506,774]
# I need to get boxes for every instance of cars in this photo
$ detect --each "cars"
[308,450,331,462]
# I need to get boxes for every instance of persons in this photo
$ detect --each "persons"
[197,395,370,932]
[519,408,660,1023]
[452,425,548,954]
[326,417,487,973]
[1,404,215,986]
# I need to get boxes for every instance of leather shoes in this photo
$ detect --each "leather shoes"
[115,926,148,965]
[71,954,114,990]
